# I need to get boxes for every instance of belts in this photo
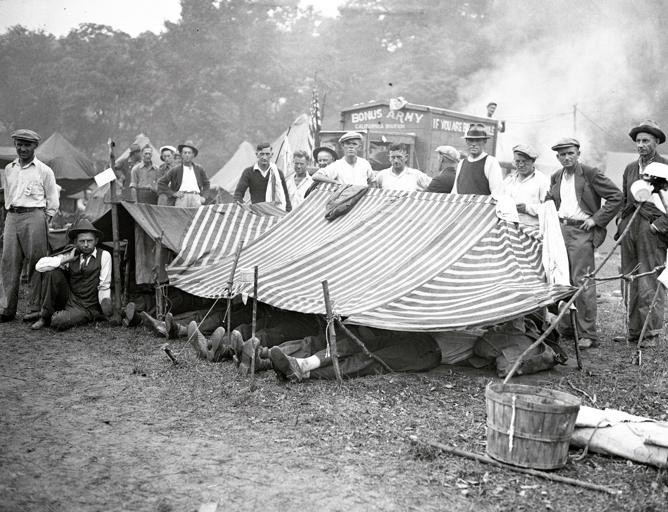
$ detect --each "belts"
[560,217,583,226]
[9,207,35,213]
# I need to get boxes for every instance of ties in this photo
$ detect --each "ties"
[82,255,90,268]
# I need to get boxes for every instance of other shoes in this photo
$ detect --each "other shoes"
[111,294,303,383]
[30,318,46,330]
[23,311,41,322]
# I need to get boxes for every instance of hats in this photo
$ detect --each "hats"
[177,140,199,158]
[312,142,339,161]
[68,218,104,243]
[461,123,490,139]
[338,131,363,144]
[435,145,461,164]
[11,128,40,143]
[160,145,177,161]
[129,142,142,152]
[512,143,540,160]
[142,143,154,153]
[551,137,581,151]
[629,120,665,146]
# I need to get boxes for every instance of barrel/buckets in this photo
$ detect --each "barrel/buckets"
[484,384,583,469]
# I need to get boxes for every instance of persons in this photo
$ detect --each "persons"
[612,118,668,349]
[29,218,114,333]
[231,130,379,209]
[112,143,211,207]
[421,143,461,194]
[376,141,429,194]
[501,143,551,203]
[1,128,60,324]
[481,100,507,134]
[449,121,504,197]
[542,135,624,351]
[121,291,527,382]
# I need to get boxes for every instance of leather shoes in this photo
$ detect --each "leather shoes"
[560,334,659,348]
